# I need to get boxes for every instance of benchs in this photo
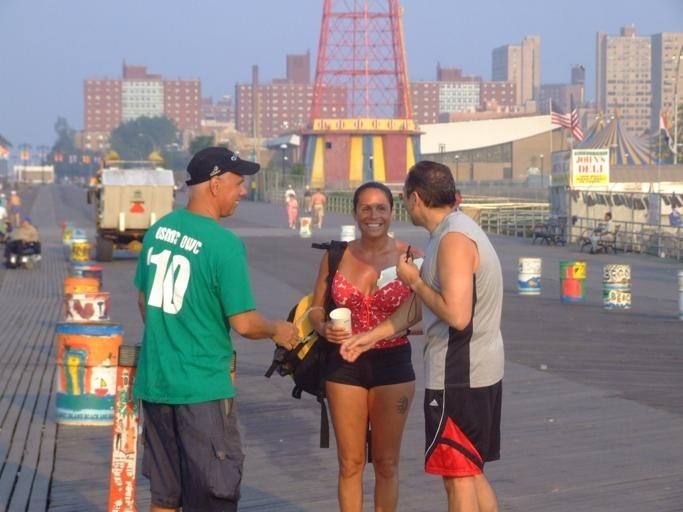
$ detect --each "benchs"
[580,224,621,254]
[532,215,566,246]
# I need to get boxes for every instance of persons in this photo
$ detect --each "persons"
[590,213,615,252]
[3,216,41,267]
[285,184,296,203]
[308,181,423,512]
[339,161,505,512]
[7,190,22,226]
[285,195,298,229]
[133,147,299,512]
[303,185,312,215]
[309,188,327,229]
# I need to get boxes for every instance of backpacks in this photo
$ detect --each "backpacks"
[265,241,347,448]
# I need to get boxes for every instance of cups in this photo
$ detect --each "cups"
[328,307,353,333]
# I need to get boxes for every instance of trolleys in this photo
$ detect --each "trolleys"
[6,226,42,268]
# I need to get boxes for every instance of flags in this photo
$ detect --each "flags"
[571,97,583,141]
[660,112,677,154]
[551,100,570,128]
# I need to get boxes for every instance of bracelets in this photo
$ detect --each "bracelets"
[402,327,411,338]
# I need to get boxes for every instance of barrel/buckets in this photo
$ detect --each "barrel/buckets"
[338,224,356,243]
[61,221,92,261]
[600,264,633,310]
[299,216,312,238]
[72,265,103,290]
[559,260,586,304]
[53,322,125,427]
[63,278,100,293]
[516,256,543,296]
[59,292,114,323]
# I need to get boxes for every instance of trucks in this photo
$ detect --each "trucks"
[92,156,175,262]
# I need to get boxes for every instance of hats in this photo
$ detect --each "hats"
[185,147,260,186]
